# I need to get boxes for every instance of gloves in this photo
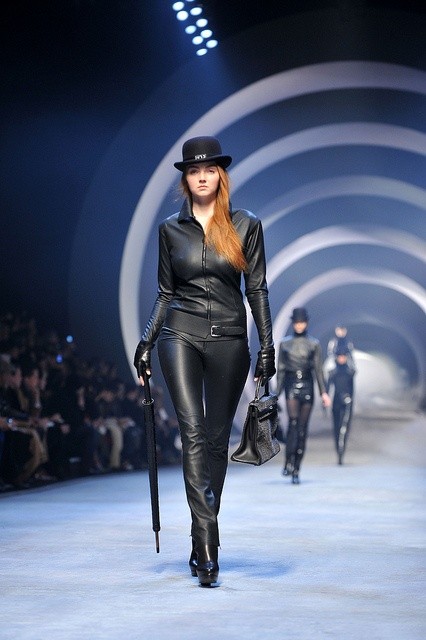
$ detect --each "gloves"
[133,341,155,378]
[254,350,275,387]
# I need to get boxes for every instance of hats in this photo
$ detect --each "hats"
[290,308,309,322]
[174,136,232,172]
[335,344,348,355]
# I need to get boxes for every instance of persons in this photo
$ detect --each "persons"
[0,309,183,490]
[133,136,275,587]
[273,306,332,484]
[323,339,355,464]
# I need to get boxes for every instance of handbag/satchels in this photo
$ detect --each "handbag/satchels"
[231,376,280,465]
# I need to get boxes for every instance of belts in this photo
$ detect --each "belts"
[164,307,246,339]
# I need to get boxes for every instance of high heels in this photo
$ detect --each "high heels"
[195,545,218,587]
[291,467,299,484]
[283,459,293,476]
[189,543,199,577]
